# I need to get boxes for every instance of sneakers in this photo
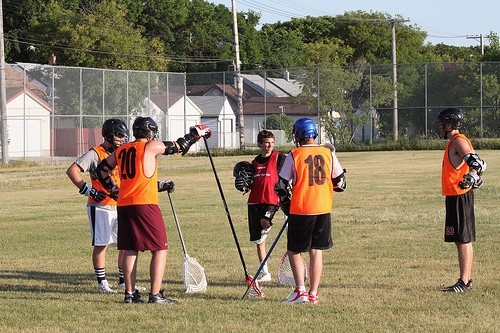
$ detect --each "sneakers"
[148,290,177,304]
[441,278,473,292]
[283,287,309,303]
[309,289,320,303]
[97,280,116,294]
[118,282,146,290]
[124,290,144,303]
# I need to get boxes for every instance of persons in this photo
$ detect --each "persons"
[436,108,486,293]
[66,119,174,295]
[95,117,212,305]
[275,118,347,304]
[233,130,287,283]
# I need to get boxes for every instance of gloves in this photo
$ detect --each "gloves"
[157,180,175,193]
[459,174,474,189]
[107,184,119,201]
[190,124,212,140]
[78,182,106,202]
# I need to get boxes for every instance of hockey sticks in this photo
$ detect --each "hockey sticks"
[165,191,209,293]
[241,142,336,301]
[203,137,264,300]
[277,207,308,287]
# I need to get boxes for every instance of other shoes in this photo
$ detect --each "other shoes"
[257,272,271,281]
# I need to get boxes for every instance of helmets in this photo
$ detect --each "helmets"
[233,161,253,182]
[132,116,158,139]
[101,118,129,148]
[435,108,463,139]
[291,117,317,143]
